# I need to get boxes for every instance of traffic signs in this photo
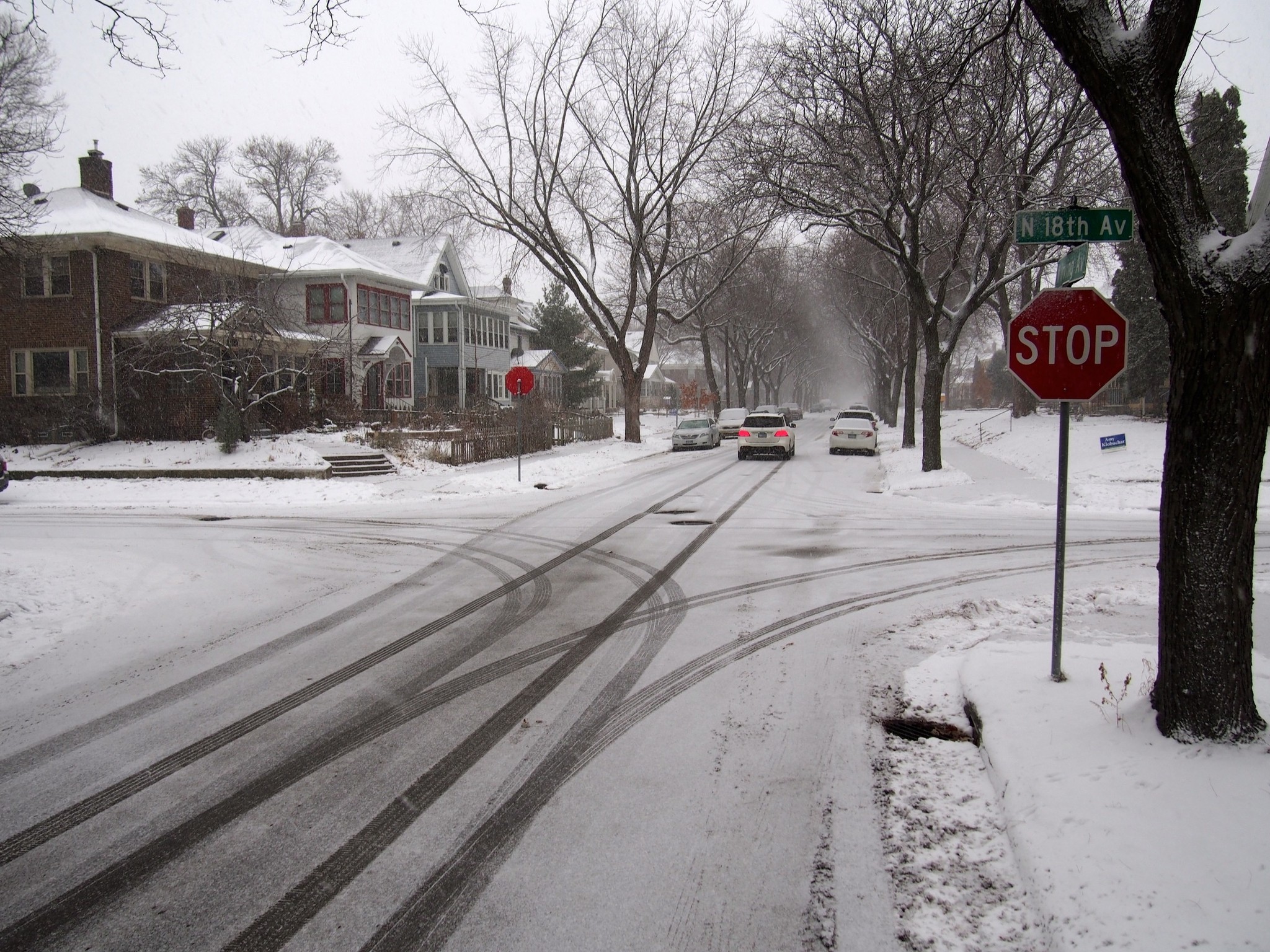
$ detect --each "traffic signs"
[1055,242,1089,288]
[1014,207,1133,245]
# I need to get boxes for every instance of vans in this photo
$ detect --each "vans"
[820,399,832,411]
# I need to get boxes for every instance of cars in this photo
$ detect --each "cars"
[829,403,880,456]
[755,403,803,423]
[831,403,839,409]
[810,402,824,413]
[672,418,720,451]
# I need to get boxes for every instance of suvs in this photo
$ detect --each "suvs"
[738,410,796,460]
[716,407,750,439]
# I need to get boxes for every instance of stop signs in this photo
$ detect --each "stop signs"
[1008,286,1129,402]
